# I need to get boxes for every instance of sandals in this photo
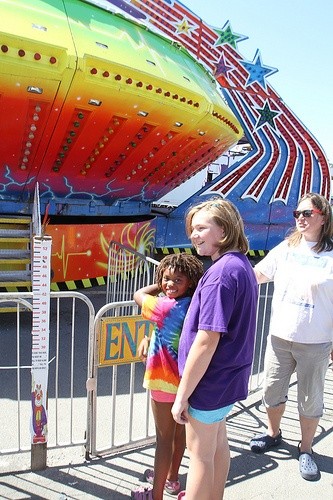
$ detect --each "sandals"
[250,428,283,453]
[145,468,180,495]
[297,441,319,481]
[130,488,162,500]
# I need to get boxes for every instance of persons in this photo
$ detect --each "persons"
[250,193,333,481]
[131,251,205,500]
[170,198,259,500]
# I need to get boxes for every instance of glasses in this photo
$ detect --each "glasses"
[293,209,322,218]
